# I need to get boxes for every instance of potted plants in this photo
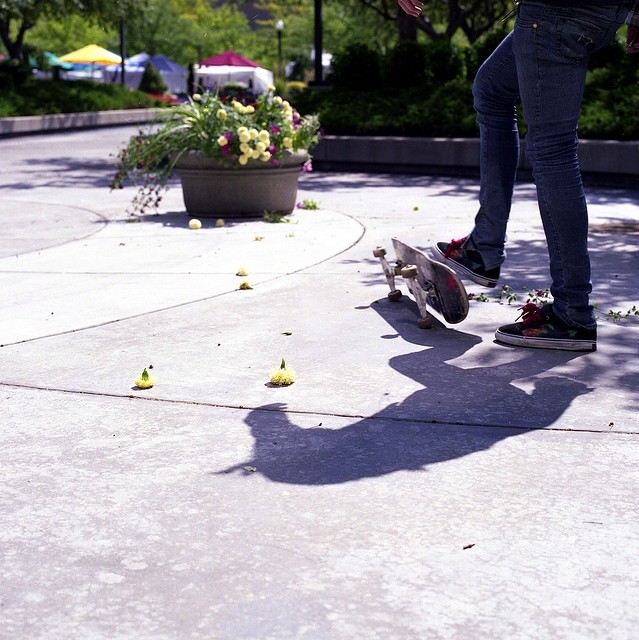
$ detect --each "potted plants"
[107,83,324,220]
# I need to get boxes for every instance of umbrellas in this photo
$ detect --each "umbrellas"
[58,42,127,79]
[196,50,263,80]
[128,51,188,75]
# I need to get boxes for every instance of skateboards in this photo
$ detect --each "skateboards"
[373,237,470,329]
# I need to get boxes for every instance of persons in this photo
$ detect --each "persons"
[396,0,630,352]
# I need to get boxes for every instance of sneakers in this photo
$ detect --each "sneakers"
[496,304,596,351]
[431,237,507,288]
